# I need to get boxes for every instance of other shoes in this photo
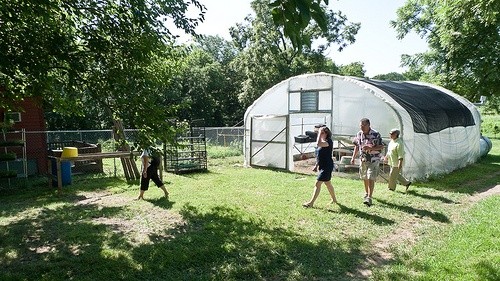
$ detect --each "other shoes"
[406,182,411,190]
[303,203,313,208]
[363,198,372,205]
[364,193,368,198]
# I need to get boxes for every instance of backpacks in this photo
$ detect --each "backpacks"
[148,147,160,168]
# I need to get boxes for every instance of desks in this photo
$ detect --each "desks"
[292,141,316,160]
[46,151,142,188]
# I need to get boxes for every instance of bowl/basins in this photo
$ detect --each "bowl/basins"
[61,147,79,159]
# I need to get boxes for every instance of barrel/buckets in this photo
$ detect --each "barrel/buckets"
[51,159,71,186]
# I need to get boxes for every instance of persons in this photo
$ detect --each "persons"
[301,127,338,209]
[133,138,171,202]
[350,118,385,205]
[312,146,321,172]
[383,128,412,193]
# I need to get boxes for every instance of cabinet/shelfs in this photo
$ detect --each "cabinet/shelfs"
[164,118,208,175]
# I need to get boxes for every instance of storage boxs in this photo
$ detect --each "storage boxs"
[294,136,310,143]
